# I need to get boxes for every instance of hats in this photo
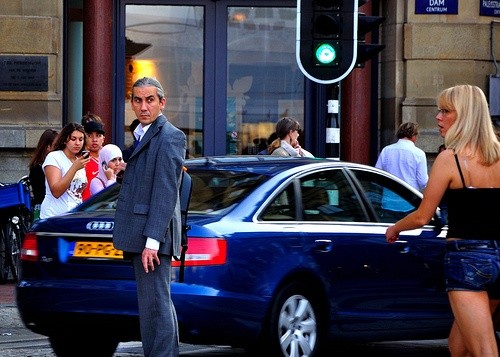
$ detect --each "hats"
[84,120,105,132]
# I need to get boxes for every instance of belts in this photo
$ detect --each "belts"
[444,243,495,251]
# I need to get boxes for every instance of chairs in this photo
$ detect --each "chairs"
[286,186,330,221]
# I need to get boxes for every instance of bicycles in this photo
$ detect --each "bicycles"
[0,176,32,285]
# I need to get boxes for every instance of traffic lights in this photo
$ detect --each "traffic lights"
[296,0,359,85]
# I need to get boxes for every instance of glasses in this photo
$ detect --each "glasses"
[438,108,456,116]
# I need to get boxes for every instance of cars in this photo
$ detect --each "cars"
[15,156,500,357]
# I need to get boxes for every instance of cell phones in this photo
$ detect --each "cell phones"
[102,161,108,170]
[81,151,90,159]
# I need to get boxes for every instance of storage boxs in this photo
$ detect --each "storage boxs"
[0,182,32,214]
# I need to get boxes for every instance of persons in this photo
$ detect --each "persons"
[113,78,187,357]
[256,117,314,158]
[28,113,140,222]
[373,122,448,225]
[386,85,500,357]
[185,129,306,158]
